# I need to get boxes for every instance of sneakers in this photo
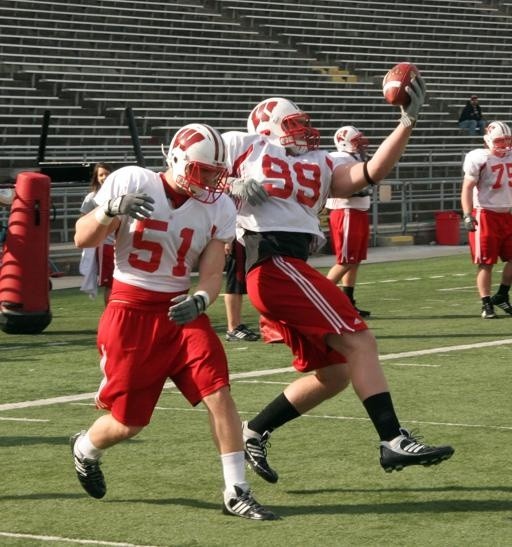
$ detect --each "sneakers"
[490,292,512,316]
[377,427,456,474]
[235,324,259,339]
[481,301,497,319]
[70,432,107,499]
[225,329,258,342]
[222,482,280,521]
[241,419,279,483]
[350,299,371,317]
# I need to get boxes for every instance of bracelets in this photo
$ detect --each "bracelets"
[193,290,210,310]
[94,201,114,228]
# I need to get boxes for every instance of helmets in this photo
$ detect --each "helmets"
[333,125,369,154]
[483,120,512,157]
[246,97,321,151]
[165,123,229,204]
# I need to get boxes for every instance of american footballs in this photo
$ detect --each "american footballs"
[383,62,419,104]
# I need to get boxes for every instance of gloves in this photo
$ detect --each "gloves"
[168,294,206,327]
[463,212,478,232]
[109,193,155,221]
[398,74,426,122]
[229,177,269,207]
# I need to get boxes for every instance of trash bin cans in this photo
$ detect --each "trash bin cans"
[434,210,461,245]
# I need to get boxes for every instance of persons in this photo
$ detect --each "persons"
[67,124,273,525]
[459,94,486,145]
[79,163,118,308]
[217,76,454,483]
[460,121,511,318]
[324,126,372,316]
[224,240,261,341]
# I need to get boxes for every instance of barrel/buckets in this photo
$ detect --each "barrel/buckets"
[435,211,464,247]
